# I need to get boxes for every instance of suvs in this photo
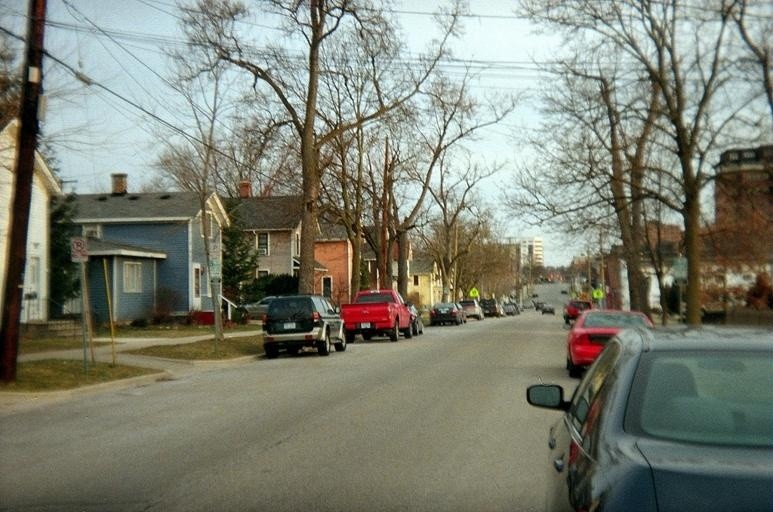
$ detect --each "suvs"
[262,293,346,359]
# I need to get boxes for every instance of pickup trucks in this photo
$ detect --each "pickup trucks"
[339,288,414,343]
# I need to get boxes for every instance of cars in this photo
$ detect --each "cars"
[564,309,655,378]
[560,287,569,295]
[237,295,284,320]
[378,303,424,336]
[429,293,555,327]
[525,325,773,512]
[562,300,592,325]
